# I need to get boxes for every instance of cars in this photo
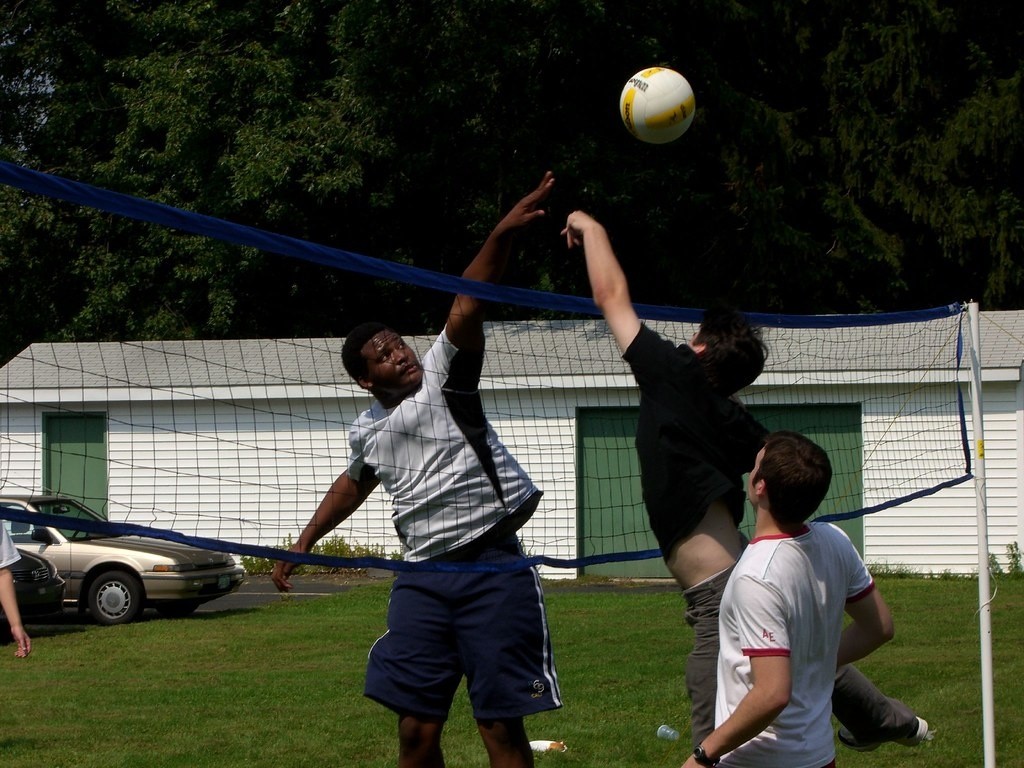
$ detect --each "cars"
[0,547,65,632]
[0,494,247,625]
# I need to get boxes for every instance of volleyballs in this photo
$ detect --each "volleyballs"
[620,66,697,144]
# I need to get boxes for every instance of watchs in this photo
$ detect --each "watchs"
[693,741,720,766]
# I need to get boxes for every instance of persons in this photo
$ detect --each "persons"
[272,170,563,768]
[0,520,31,659]
[561,211,928,768]
[679,431,895,768]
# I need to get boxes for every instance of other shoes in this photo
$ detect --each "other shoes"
[838,716,928,752]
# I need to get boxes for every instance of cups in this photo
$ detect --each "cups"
[657,724,679,743]
